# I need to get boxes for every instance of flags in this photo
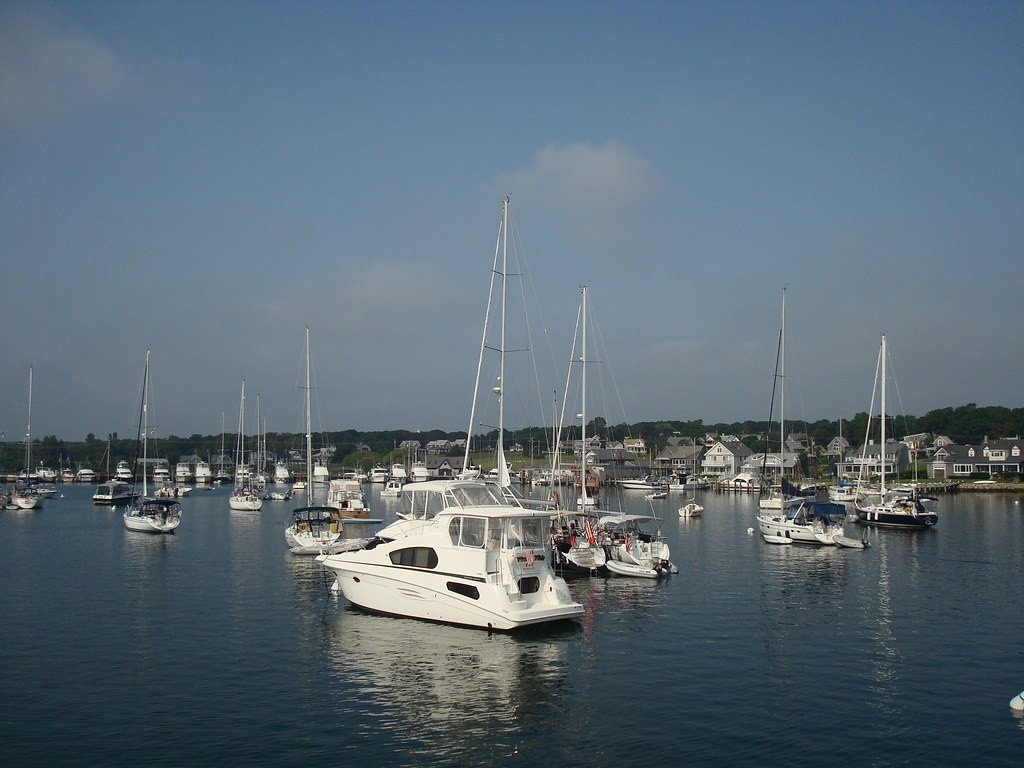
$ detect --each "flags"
[585,519,595,545]
[525,550,535,565]
[624,531,633,553]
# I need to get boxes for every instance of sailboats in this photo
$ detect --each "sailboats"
[0,194,536,545]
[284,325,344,549]
[854,332,939,529]
[758,286,816,509]
[538,285,679,579]
[11,365,41,510]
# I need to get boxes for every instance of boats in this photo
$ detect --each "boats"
[757,512,844,545]
[379,480,404,500]
[153,485,192,497]
[315,501,586,631]
[123,496,181,531]
[678,504,704,516]
[325,479,371,522]
[92,480,140,505]
[828,485,865,500]
[229,494,263,510]
